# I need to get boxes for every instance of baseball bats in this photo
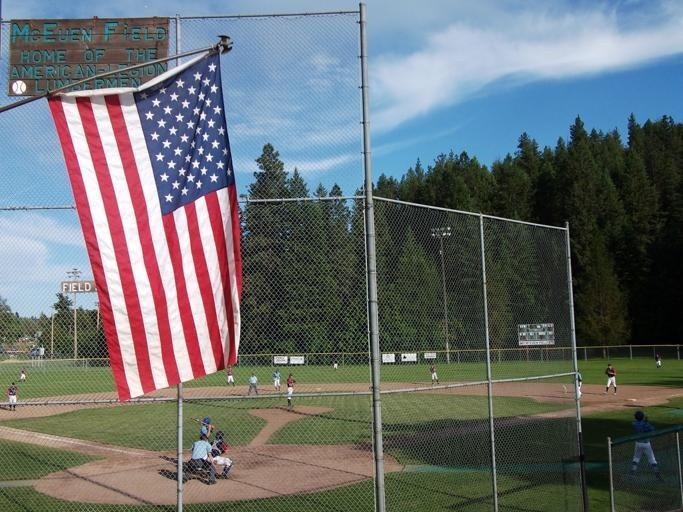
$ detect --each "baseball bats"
[194,418,208,426]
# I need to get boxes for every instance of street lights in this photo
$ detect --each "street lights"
[65,266,81,360]
[93,300,101,336]
[45,304,55,357]
[429,223,455,366]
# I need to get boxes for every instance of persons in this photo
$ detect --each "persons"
[225,367,235,387]
[654,353,661,369]
[6,382,19,411]
[603,363,617,395]
[577,369,583,399]
[199,416,215,442]
[272,370,281,392]
[181,433,216,485]
[206,430,233,479]
[247,372,258,395]
[286,374,295,406]
[16,370,25,382]
[628,410,663,481]
[429,363,439,385]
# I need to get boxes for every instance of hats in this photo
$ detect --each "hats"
[200,434,208,439]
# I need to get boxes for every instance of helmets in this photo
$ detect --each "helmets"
[215,431,224,438]
[634,411,644,416]
[204,417,209,423]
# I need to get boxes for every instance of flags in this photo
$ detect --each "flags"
[45,48,241,405]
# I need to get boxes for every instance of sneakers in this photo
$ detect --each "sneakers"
[222,471,229,479]
[207,481,216,485]
